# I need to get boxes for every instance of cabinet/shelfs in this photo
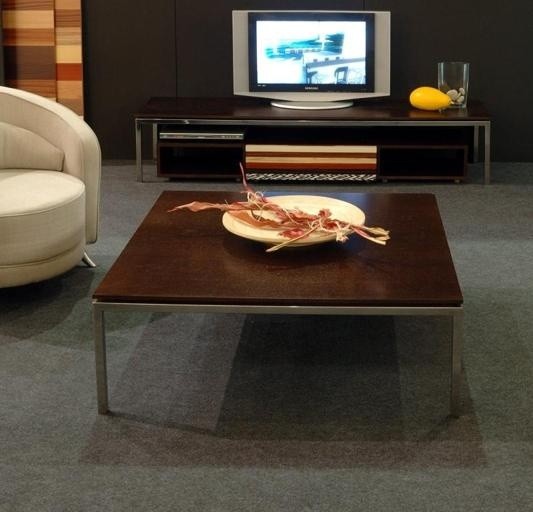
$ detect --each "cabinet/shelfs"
[132,96,491,185]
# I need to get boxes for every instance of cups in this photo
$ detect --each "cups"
[437,61,470,111]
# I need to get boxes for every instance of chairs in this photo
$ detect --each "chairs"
[0,85,103,290]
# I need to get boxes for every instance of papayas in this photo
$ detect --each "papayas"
[410,86,451,111]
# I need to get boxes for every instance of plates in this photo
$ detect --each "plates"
[222,195,366,247]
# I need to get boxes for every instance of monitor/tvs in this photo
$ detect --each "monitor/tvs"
[232,9,391,110]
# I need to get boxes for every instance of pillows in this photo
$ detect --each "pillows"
[1,123,65,171]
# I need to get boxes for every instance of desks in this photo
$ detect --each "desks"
[92,190,465,418]
